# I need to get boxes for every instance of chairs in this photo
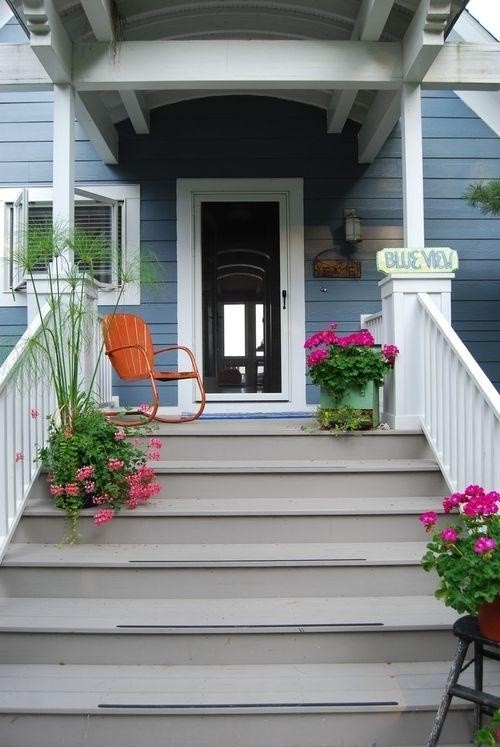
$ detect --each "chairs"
[99,312,208,427]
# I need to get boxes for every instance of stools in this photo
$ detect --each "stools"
[426,616,500,746]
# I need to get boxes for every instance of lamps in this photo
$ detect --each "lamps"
[335,206,364,245]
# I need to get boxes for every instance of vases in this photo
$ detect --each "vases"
[317,381,379,428]
[477,591,500,642]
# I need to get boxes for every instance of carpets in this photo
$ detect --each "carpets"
[177,410,318,419]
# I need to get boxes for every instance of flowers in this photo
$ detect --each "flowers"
[302,319,400,389]
[419,481,500,616]
[11,392,166,549]
